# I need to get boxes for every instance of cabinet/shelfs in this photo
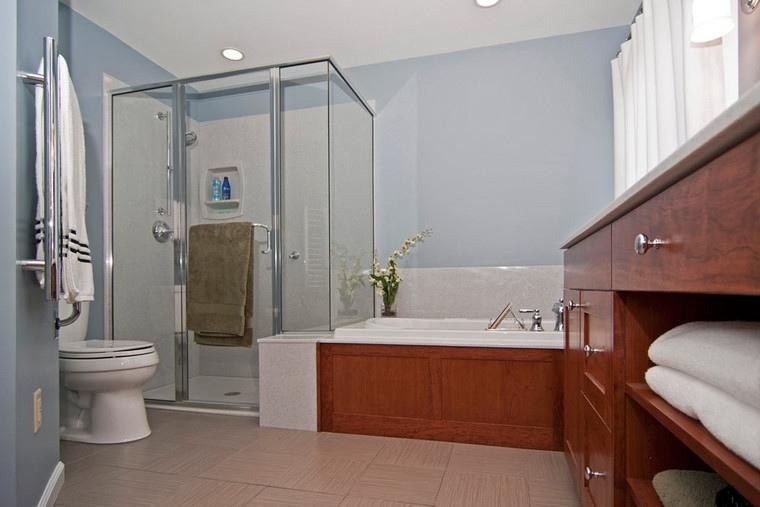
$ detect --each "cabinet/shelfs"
[560,220,614,506]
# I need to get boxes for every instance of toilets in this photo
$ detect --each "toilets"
[59,300,160,445]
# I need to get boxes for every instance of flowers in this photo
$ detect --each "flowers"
[367,226,435,316]
[331,239,366,308]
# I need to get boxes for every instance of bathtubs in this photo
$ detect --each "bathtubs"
[332,315,566,349]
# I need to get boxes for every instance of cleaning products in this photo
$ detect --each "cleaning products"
[212,175,220,201]
[221,175,230,198]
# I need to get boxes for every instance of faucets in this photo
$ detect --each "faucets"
[552,299,562,318]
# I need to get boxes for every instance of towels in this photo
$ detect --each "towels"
[32,52,96,304]
[185,220,256,348]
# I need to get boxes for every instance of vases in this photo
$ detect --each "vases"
[380,295,398,317]
